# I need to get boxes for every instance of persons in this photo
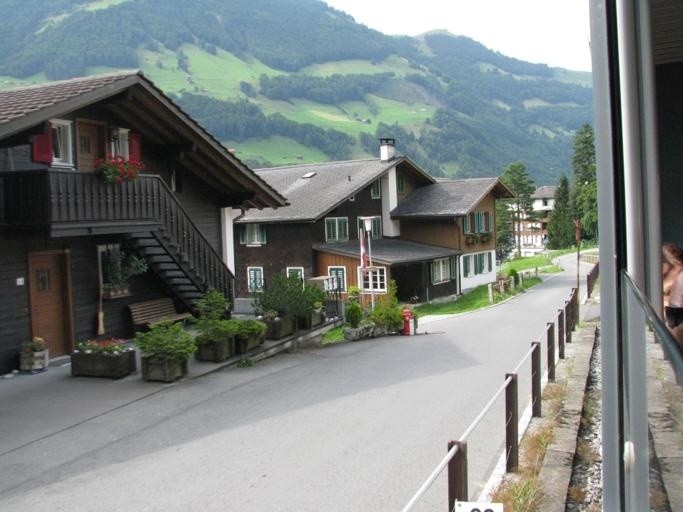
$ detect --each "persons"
[662,242,683,349]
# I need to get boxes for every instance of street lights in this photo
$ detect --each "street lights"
[361,215,378,311]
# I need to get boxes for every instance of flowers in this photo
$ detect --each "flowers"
[97,154,144,184]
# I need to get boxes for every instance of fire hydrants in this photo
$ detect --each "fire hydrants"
[401,309,412,335]
